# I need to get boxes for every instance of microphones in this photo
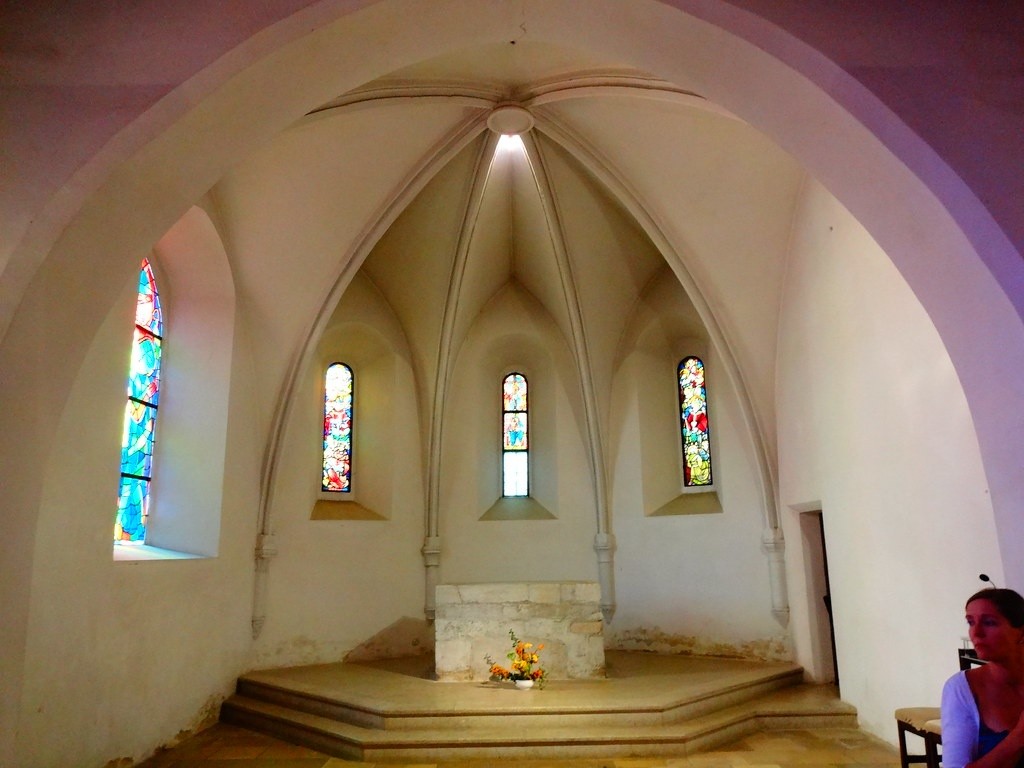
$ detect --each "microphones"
[980,574,996,589]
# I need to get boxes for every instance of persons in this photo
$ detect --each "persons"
[940,588,1024,768]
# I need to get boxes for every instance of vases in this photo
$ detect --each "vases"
[515,680,533,691]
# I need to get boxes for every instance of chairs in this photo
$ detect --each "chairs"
[895,648,989,768]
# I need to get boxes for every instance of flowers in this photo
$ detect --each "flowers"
[481,628,549,691]
